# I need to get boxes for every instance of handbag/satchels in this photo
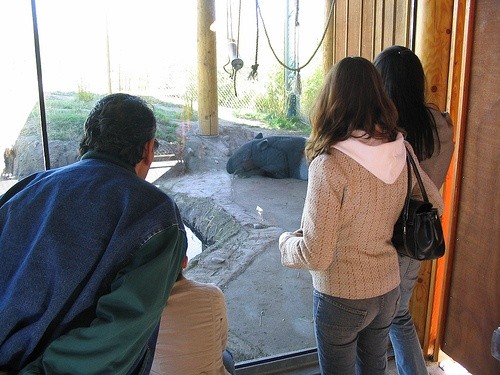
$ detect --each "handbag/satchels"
[392,145,446,261]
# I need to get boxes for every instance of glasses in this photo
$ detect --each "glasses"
[153,139,159,153]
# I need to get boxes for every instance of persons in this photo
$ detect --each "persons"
[146,252,237,375]
[1,93,186,375]
[278,52,443,375]
[342,45,456,375]
